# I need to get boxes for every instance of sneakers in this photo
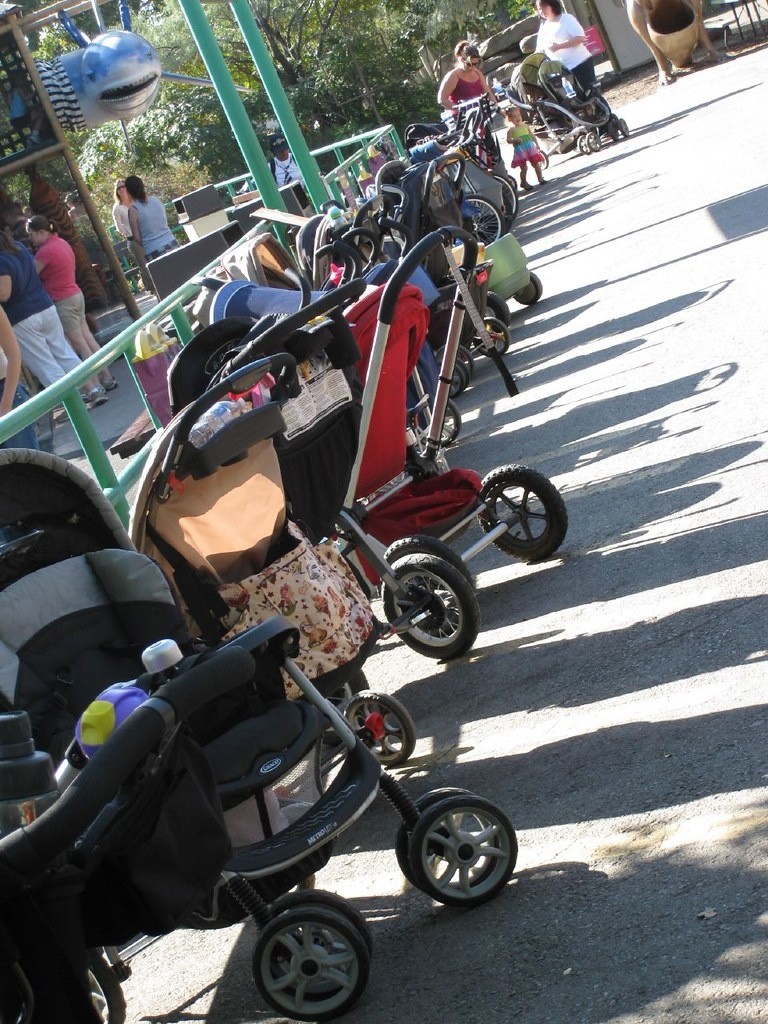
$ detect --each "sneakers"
[56,396,92,423]
[89,387,108,406]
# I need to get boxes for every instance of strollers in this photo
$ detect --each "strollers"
[500,50,633,156]
[0,78,572,1024]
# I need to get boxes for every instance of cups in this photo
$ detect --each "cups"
[440,110,456,131]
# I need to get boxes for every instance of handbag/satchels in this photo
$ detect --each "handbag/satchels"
[206,521,374,699]
[481,232,531,301]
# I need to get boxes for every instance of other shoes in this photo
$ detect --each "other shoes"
[521,182,534,190]
[539,180,548,184]
[101,376,119,390]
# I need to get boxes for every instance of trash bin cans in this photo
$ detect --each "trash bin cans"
[144,231,228,300]
[226,181,315,239]
[171,184,231,235]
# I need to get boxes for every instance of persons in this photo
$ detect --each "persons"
[438,41,506,148]
[0,201,36,256]
[534,0,611,115]
[65,193,86,220]
[505,106,548,190]
[264,133,304,187]
[8,69,45,144]
[0,305,41,451]
[22,205,32,217]
[112,179,156,295]
[0,229,109,422]
[25,214,119,390]
[125,175,180,262]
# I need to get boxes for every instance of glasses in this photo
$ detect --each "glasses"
[463,60,479,67]
[117,185,125,191]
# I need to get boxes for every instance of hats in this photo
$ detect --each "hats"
[270,133,289,153]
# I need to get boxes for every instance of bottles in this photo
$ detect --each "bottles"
[141,638,185,695]
[189,398,247,449]
[493,78,504,96]
[562,77,574,98]
[0,710,62,839]
[329,206,347,230]
